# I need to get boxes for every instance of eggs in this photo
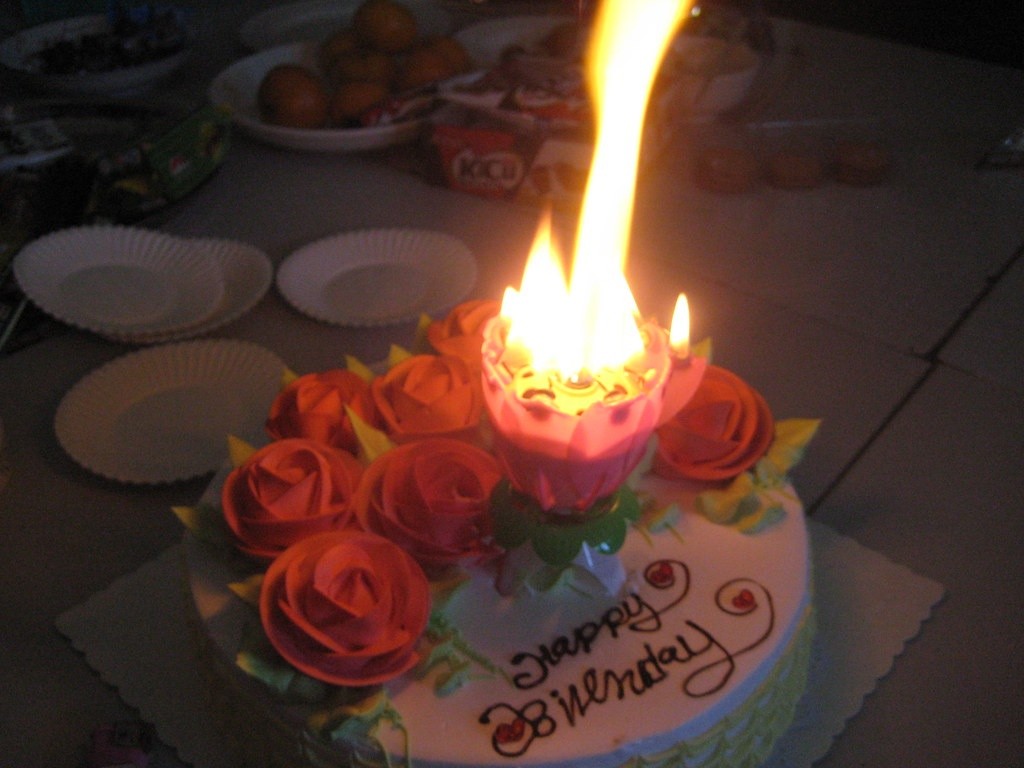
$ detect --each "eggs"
[258,0,466,128]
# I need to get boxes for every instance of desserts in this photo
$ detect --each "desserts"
[693,140,890,190]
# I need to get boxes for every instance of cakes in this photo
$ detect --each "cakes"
[168,297,823,768]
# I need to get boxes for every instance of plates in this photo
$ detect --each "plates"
[14,223,224,334]
[238,0,454,54]
[276,226,465,329]
[208,42,481,148]
[55,340,300,485]
[456,15,576,72]
[100,235,273,344]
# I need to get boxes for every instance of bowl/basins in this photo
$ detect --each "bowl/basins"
[0,12,194,104]
[678,37,760,123]
